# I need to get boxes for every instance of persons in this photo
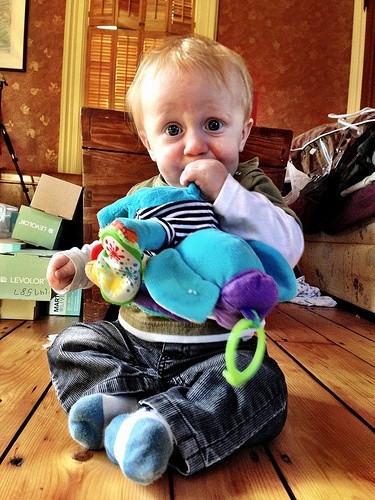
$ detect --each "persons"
[45,34,304,486]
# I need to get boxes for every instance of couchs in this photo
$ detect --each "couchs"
[297,221,375,314]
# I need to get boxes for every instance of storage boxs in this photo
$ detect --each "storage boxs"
[0,173,85,319]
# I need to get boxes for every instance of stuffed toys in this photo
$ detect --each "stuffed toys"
[85,183,297,387]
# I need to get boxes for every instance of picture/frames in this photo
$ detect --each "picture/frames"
[0,0,29,72]
